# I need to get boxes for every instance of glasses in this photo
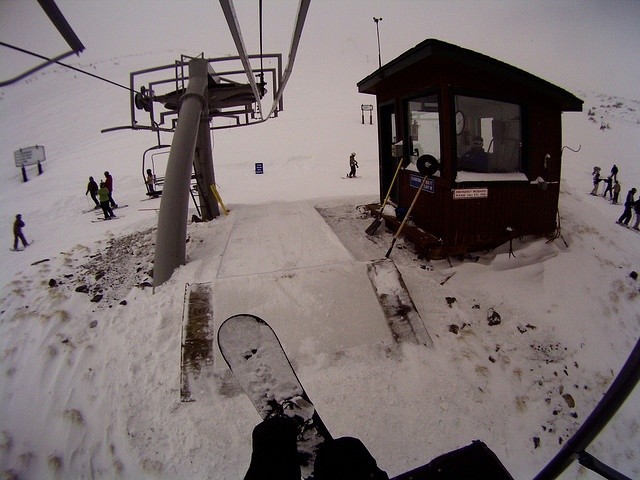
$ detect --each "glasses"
[472,141,483,147]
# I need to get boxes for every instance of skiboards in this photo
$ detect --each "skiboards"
[94,204,129,216]
[83,206,100,214]
[91,215,124,222]
[9,240,35,252]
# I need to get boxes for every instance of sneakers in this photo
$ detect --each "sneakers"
[265,394,315,433]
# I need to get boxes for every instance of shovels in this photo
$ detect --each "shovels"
[365,157,403,234]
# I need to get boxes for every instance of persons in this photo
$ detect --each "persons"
[619,188,636,226]
[461,135,489,170]
[590,170,603,196]
[145,170,155,192]
[12,214,30,249]
[99,182,114,220]
[348,154,358,178]
[610,165,618,182]
[86,176,100,209]
[603,176,613,199]
[103,171,117,208]
[592,167,601,177]
[632,196,640,232]
[612,180,620,203]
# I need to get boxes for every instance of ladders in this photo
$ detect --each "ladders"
[189,162,212,222]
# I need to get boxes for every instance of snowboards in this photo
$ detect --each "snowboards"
[612,202,623,205]
[218,313,334,480]
[616,222,640,234]
